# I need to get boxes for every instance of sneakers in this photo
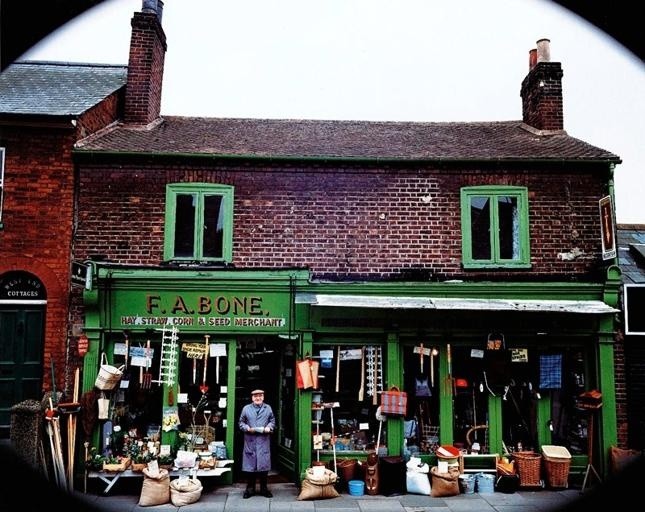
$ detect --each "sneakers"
[243,490,256,498]
[259,488,273,498]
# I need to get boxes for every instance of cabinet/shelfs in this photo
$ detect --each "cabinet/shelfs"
[88,459,235,495]
[459,453,500,478]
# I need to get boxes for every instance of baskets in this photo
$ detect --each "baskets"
[512,452,542,485]
[96,352,125,391]
[544,457,570,486]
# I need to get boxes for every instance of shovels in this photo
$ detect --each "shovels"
[119,335,131,388]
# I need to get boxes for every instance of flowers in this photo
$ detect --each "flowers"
[162,385,209,452]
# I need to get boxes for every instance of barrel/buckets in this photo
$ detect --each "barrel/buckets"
[348,480,365,496]
[459,474,476,493]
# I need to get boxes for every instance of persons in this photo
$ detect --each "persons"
[238,390,276,499]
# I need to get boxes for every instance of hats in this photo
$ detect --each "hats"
[252,389,263,395]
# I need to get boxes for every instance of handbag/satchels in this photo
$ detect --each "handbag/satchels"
[380,387,407,417]
